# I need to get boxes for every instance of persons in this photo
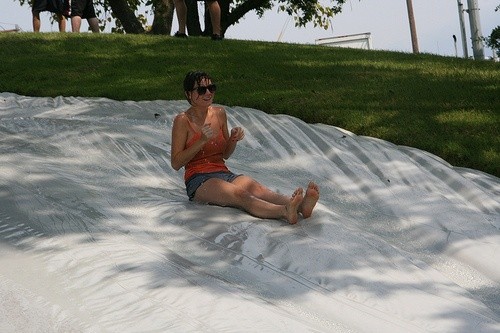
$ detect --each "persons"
[172,0,223,40]
[70,0,100,34]
[30,0,66,33]
[170,70,321,226]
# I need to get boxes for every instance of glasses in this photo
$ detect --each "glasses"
[194,84,217,95]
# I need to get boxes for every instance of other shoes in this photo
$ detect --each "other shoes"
[174,31,187,38]
[211,33,220,40]
